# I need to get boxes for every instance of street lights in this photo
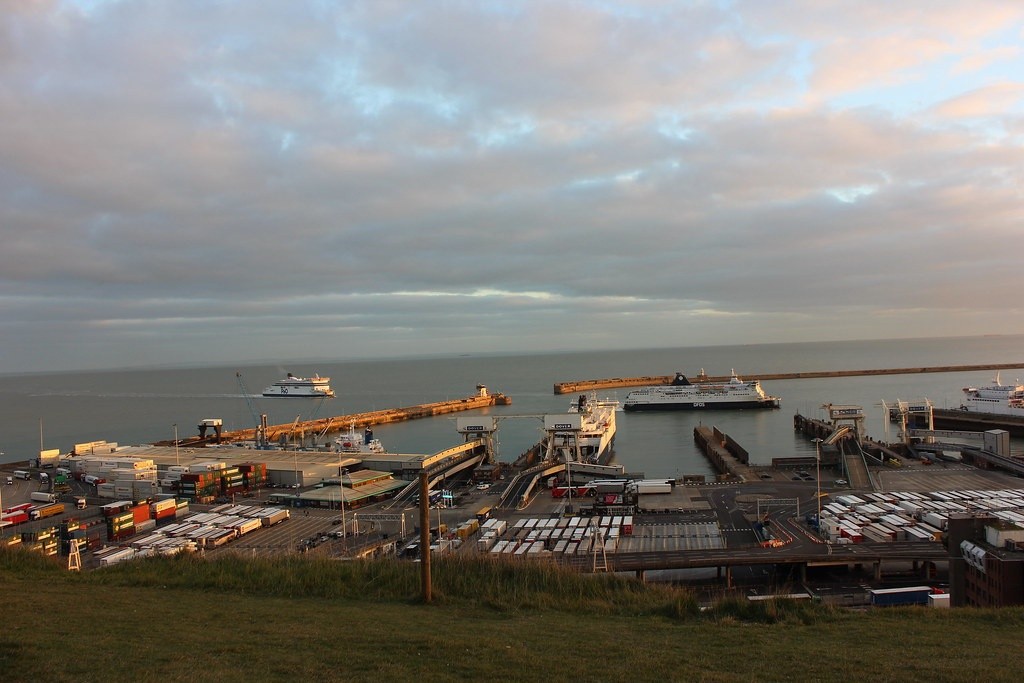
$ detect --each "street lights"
[809,437,824,536]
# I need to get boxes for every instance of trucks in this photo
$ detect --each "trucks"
[80,473,98,484]
[0,502,64,529]
[13,471,32,480]
[56,468,72,479]
[7,476,13,485]
[91,503,291,569]
[73,495,87,510]
[31,491,55,503]
[869,584,944,607]
[40,472,48,484]
[585,479,676,494]
[745,592,822,607]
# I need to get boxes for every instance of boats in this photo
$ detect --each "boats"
[544,388,619,466]
[1010,398,1023,405]
[699,385,711,390]
[712,385,725,390]
[262,372,337,398]
[336,417,384,454]
[622,367,781,411]
[959,370,1024,418]
